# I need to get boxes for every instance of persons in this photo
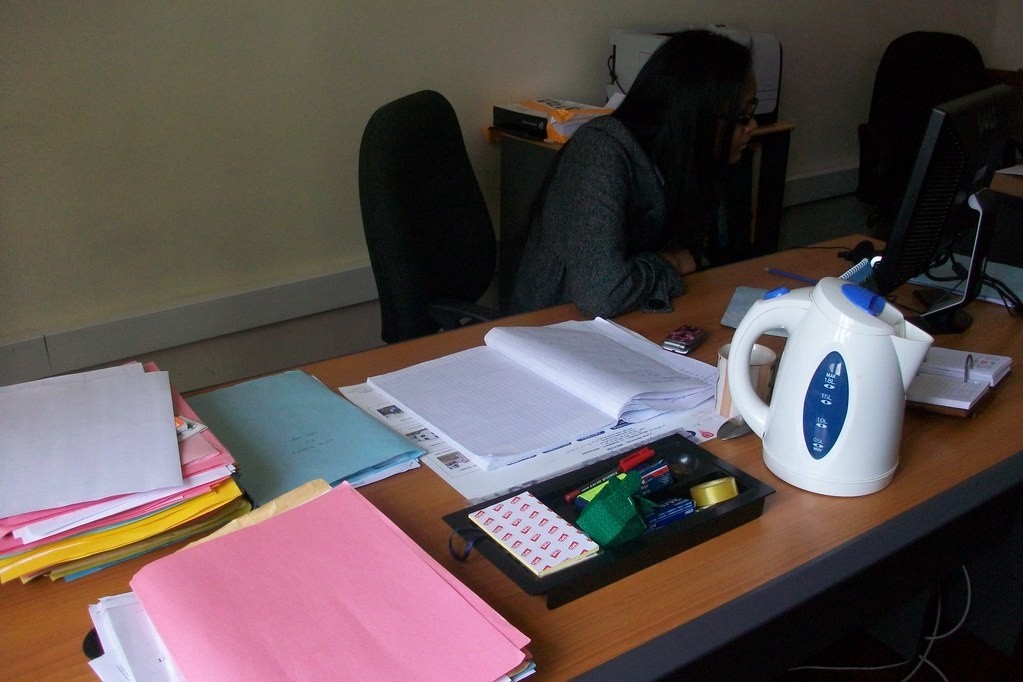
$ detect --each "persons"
[507,30,759,319]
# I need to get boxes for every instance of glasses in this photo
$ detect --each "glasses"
[731,97,760,125]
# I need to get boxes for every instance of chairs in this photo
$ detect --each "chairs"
[855,31,994,245]
[358,89,509,344]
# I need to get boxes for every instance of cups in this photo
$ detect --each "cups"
[712,342,777,419]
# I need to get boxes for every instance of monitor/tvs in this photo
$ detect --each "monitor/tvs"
[875,82,1013,335]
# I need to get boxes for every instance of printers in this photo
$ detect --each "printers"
[605,24,783,128]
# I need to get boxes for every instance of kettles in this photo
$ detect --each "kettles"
[728,277,935,498]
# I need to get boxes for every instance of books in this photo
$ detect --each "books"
[367,326,709,471]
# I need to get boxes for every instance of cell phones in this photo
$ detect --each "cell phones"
[663,324,707,355]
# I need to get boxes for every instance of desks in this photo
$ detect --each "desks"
[0,232,1023,682]
[500,117,797,310]
